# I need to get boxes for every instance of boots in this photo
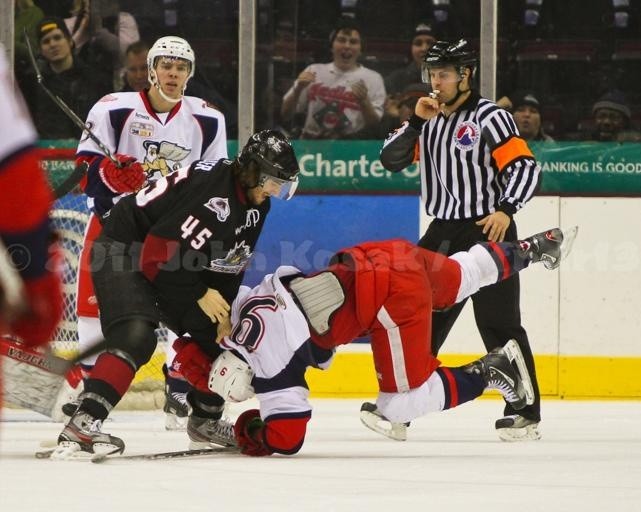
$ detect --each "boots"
[512,224,581,272]
[459,338,536,411]
[187,387,235,456]
[33,399,126,464]
[61,375,116,429]
[160,361,193,432]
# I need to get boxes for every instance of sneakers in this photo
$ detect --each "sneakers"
[493,411,545,442]
[359,401,411,441]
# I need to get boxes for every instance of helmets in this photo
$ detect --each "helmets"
[146,36,196,91]
[423,40,480,80]
[207,349,256,404]
[238,128,300,200]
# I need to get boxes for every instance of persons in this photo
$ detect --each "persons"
[355,32,543,447]
[12,0,641,144]
[42,123,300,464]
[72,32,231,434]
[0,45,65,349]
[169,225,580,457]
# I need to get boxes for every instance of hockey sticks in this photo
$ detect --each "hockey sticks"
[52,160,89,202]
[23,26,121,168]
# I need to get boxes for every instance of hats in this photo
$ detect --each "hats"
[410,21,440,43]
[395,83,432,109]
[508,88,543,121]
[591,88,633,123]
[34,16,76,54]
[327,10,366,47]
